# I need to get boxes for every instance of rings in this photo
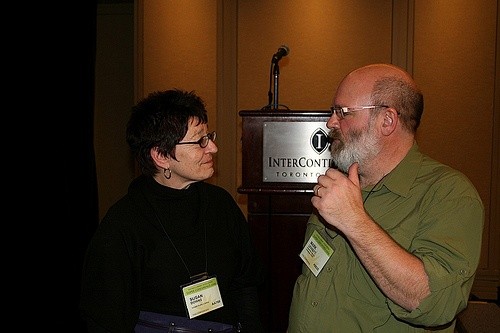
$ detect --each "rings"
[314,185,324,198]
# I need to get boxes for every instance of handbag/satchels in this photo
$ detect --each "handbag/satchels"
[133,311,240,333]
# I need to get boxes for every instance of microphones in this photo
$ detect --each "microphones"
[271,44,290,64]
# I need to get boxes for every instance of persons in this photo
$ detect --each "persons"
[72,87,274,333]
[277,63,486,333]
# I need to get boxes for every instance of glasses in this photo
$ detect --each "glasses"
[331,104,391,118]
[172,130,216,149]
